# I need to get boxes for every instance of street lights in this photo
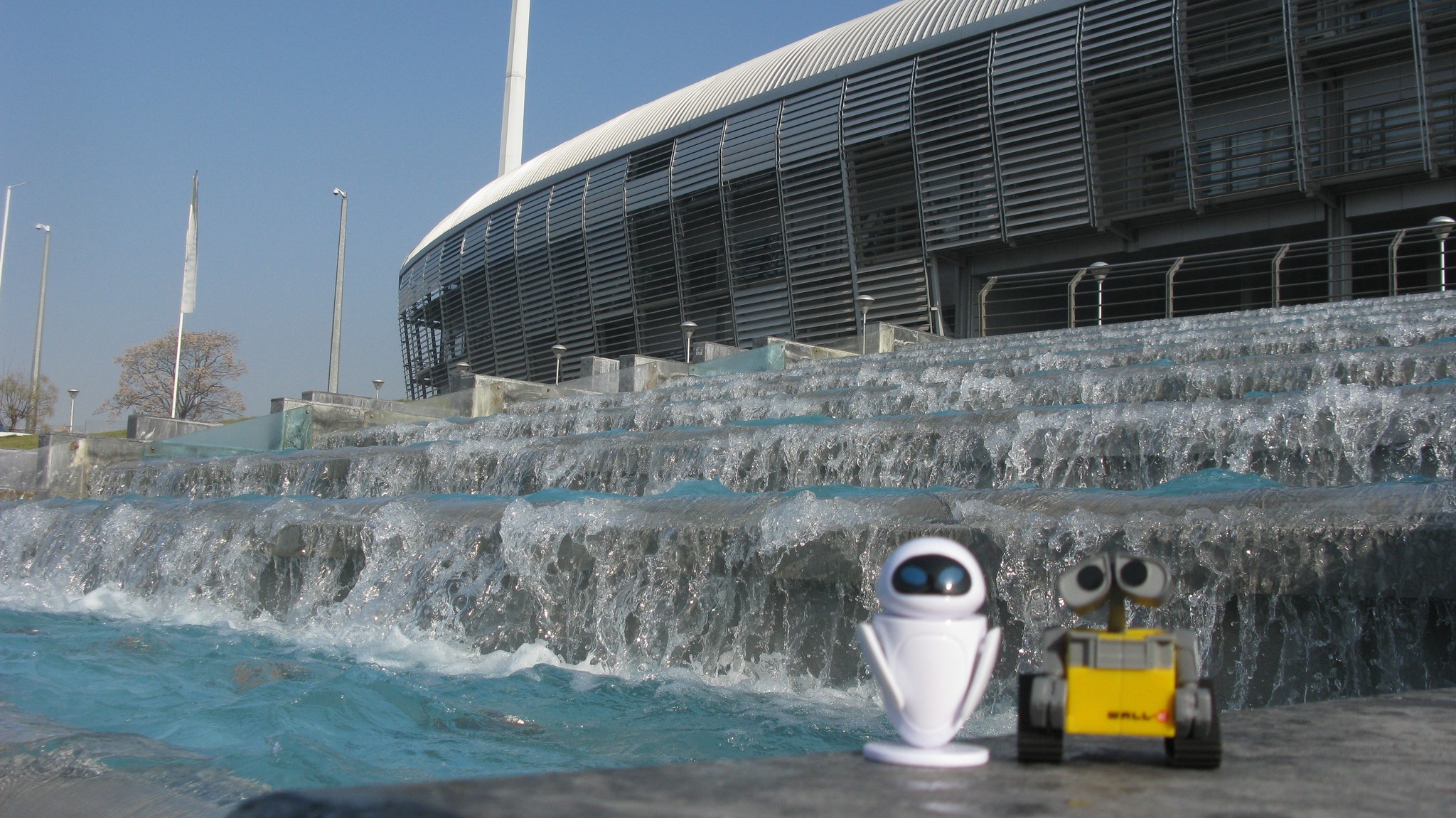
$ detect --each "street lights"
[551,343,565,382]
[853,295,874,353]
[456,361,470,374]
[24,223,50,432]
[326,188,347,392]
[67,389,79,434]
[681,322,698,364]
[1085,261,1109,328]
[0,181,28,277]
[371,379,384,396]
[1428,215,1454,290]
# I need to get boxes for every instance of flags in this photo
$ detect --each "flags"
[181,170,199,317]
